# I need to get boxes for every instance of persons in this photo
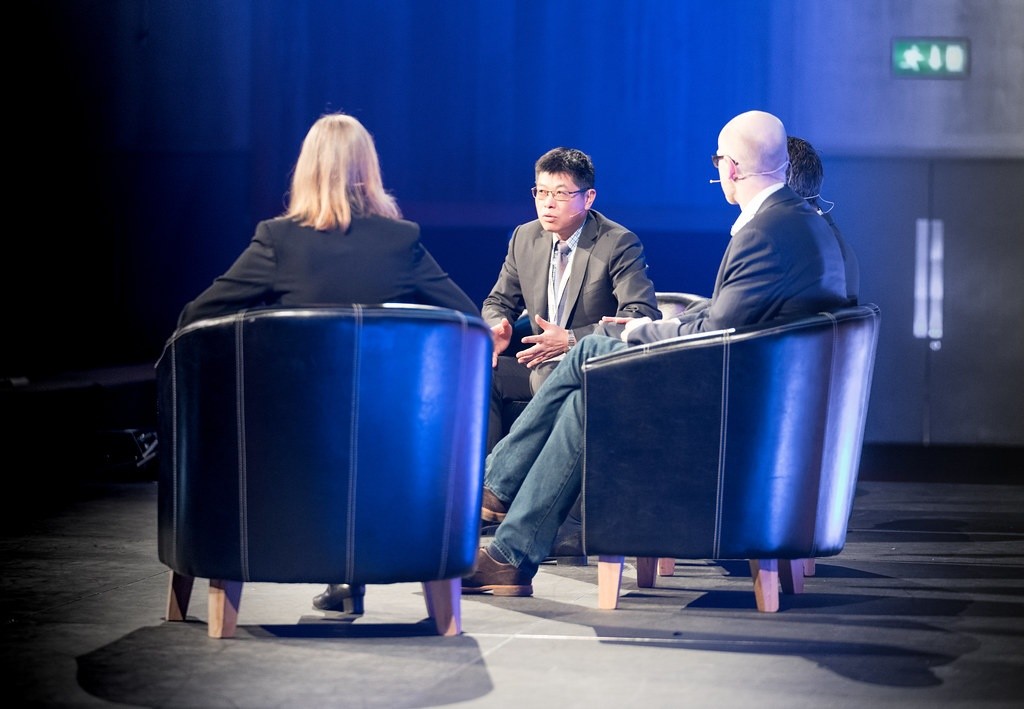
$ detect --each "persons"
[480,148,662,451]
[458,111,846,595]
[542,136,860,566]
[130,113,479,615]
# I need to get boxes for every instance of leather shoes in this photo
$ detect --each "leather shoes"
[541,514,587,567]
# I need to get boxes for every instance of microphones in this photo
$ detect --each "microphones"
[709,179,720,183]
[570,210,584,219]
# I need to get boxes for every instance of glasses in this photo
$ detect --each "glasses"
[712,156,738,168]
[531,187,591,201]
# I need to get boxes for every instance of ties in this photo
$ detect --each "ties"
[554,242,570,298]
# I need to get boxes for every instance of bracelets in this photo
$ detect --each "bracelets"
[568,331,575,351]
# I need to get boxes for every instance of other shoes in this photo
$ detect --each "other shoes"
[460,546,534,596]
[480,487,507,523]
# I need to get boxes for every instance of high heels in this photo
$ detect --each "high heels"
[313,582,366,614]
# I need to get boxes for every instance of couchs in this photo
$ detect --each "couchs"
[507,291,881,614]
[152,301,496,640]
